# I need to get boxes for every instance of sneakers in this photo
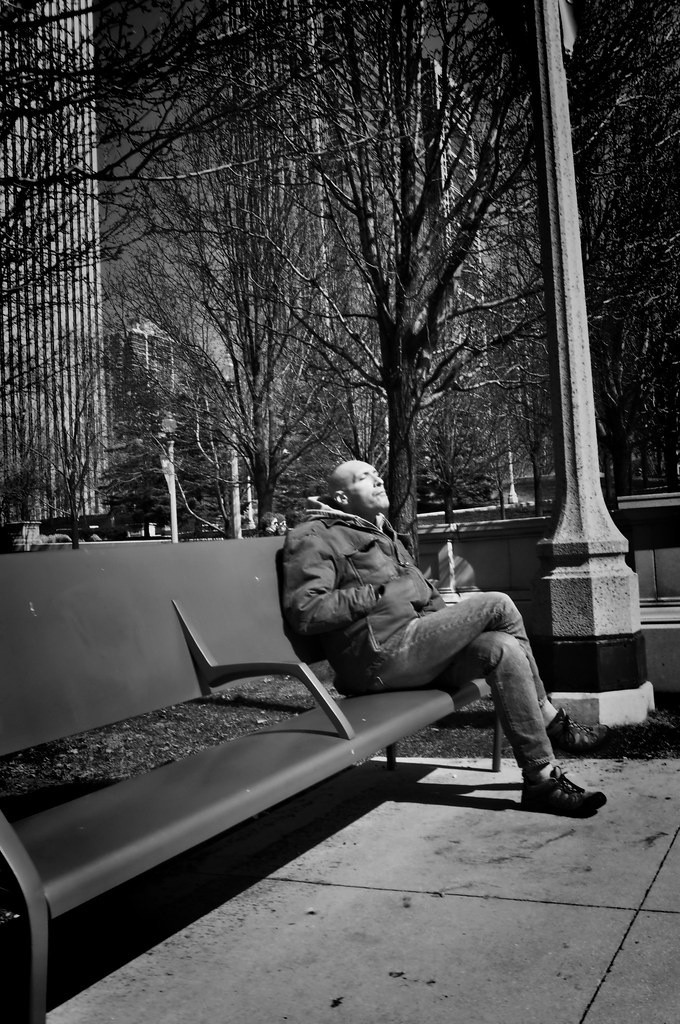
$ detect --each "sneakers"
[546,707,611,753]
[522,767,607,814]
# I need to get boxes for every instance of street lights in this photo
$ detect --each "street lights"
[162,410,179,544]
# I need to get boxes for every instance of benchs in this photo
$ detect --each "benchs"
[0,522,523,1024]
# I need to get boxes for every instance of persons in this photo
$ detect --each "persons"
[258,512,288,538]
[281,459,611,816]
